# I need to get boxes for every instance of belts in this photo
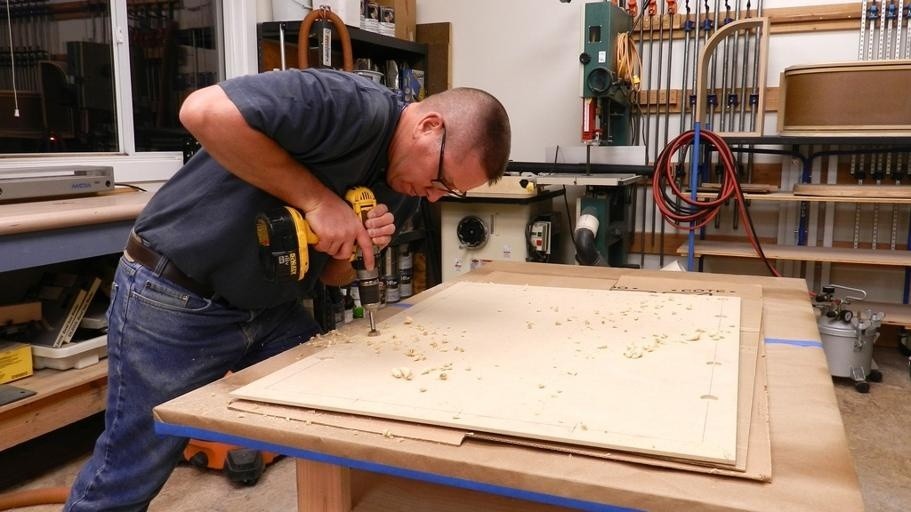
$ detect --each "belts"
[125,230,230,308]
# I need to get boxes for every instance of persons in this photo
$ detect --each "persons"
[63,64,513,512]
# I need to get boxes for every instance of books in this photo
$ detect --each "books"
[15,285,87,351]
[55,273,104,344]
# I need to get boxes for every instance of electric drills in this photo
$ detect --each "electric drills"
[256,187,381,332]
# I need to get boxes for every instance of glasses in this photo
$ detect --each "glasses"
[430,119,467,199]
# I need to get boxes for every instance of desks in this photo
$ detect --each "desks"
[153,260,867,510]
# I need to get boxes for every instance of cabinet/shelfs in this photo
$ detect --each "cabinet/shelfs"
[677,129,911,378]
[253,18,444,336]
